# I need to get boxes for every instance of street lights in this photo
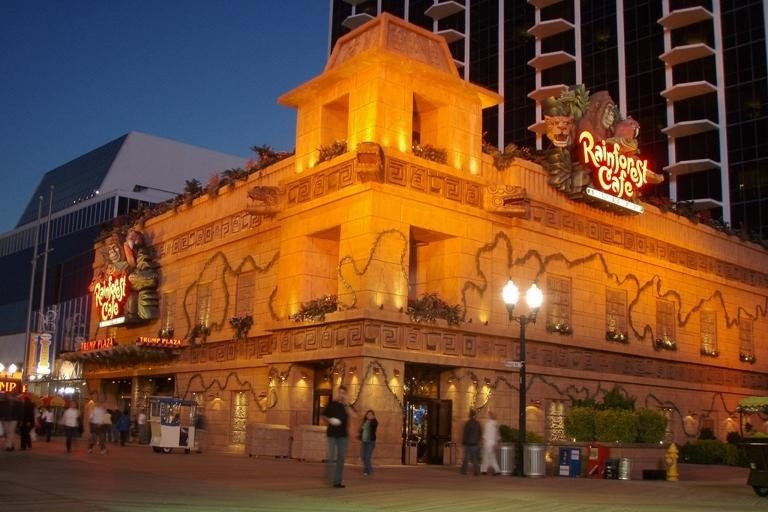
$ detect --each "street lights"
[501,280,542,476]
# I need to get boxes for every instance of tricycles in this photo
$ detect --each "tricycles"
[739,397,768,497]
[146,395,197,453]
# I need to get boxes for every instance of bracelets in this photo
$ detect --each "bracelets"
[344,404,348,407]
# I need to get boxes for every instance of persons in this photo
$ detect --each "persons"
[359,410,378,477]
[459,410,483,476]
[480,411,502,476]
[0,391,146,455]
[322,386,358,488]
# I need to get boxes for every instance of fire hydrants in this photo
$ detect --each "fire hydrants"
[666,443,680,481]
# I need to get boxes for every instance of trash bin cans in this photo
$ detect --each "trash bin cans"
[522,441,546,478]
[496,441,517,474]
[442,441,455,464]
[404,440,417,465]
